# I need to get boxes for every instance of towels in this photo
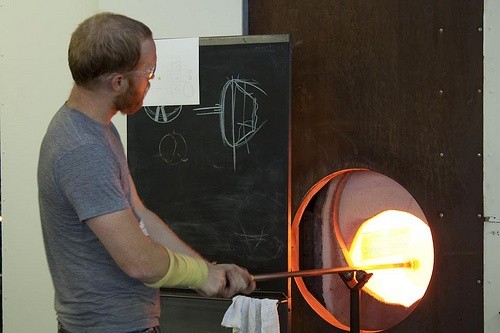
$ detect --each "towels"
[220,295,281,333]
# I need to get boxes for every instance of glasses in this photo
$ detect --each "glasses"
[104,63,156,81]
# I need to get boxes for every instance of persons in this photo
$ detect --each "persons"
[35,10,258,332]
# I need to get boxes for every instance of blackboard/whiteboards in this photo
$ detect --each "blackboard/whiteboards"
[119,35,294,300]
